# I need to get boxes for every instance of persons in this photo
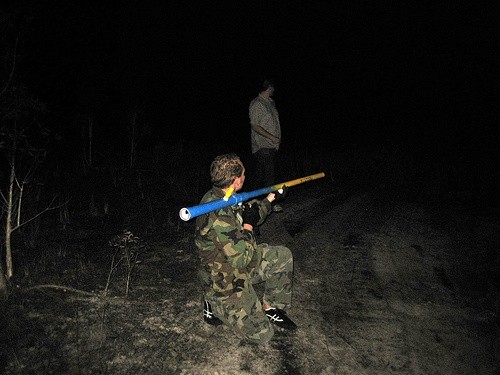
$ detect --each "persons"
[194,153,298,344]
[249,76,284,212]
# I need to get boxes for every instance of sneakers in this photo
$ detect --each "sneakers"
[272,204,284,212]
[203,299,224,326]
[265,308,298,330]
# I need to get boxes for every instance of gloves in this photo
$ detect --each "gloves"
[241,202,260,229]
[271,184,288,199]
